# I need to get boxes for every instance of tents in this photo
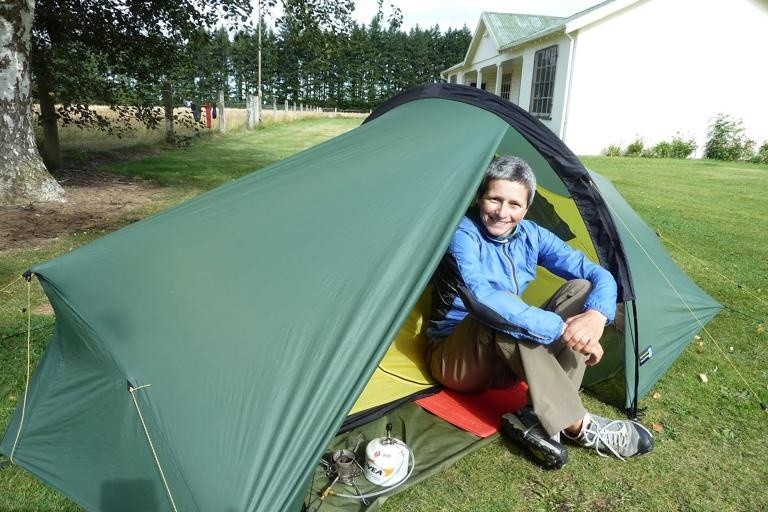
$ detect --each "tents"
[1,83,724,512]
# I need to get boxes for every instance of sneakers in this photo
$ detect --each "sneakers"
[561,412,655,460]
[501,404,568,471]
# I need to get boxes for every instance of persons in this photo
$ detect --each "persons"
[423,155,654,470]
